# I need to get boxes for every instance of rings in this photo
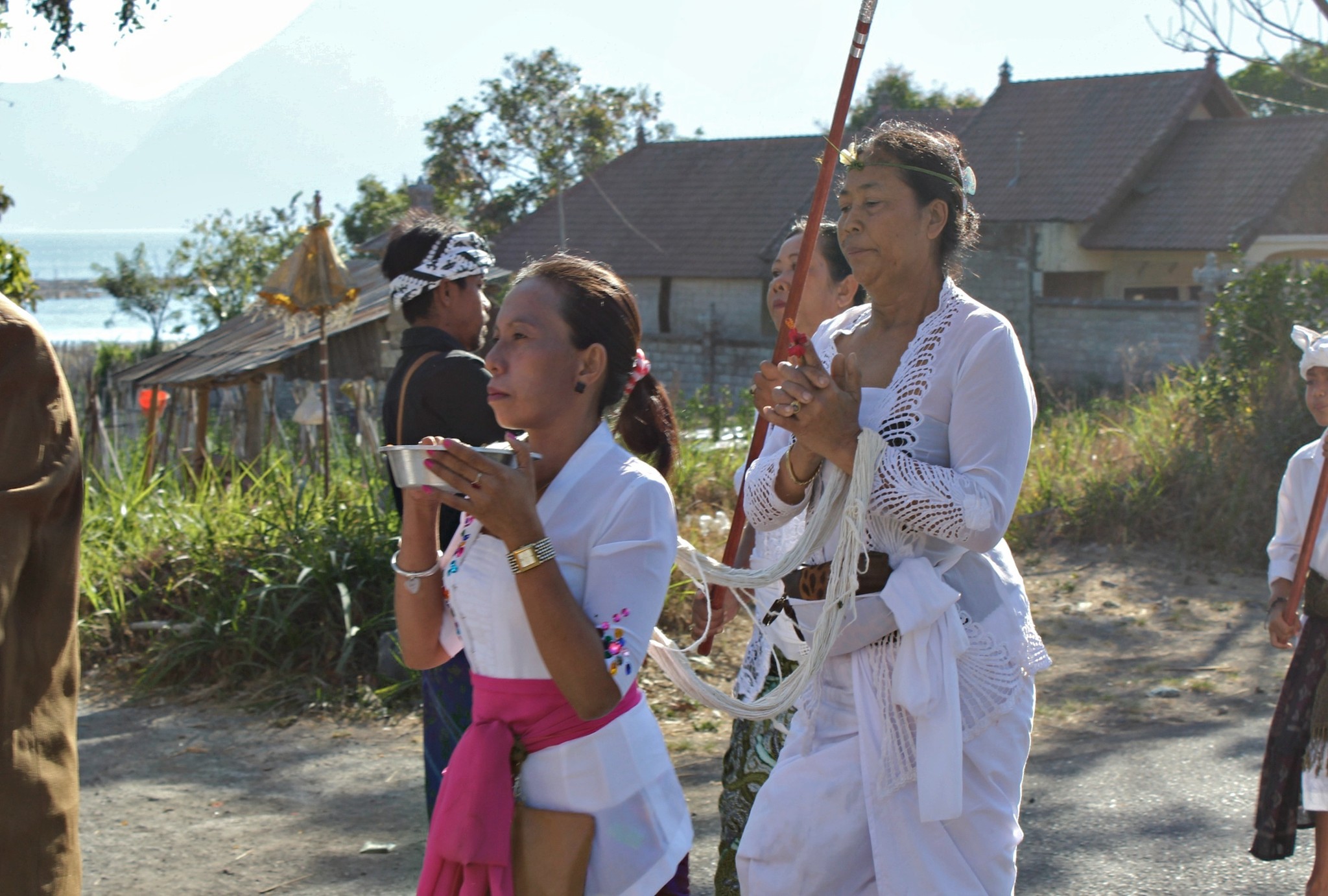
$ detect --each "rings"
[790,399,801,414]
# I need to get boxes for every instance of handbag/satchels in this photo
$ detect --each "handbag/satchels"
[512,734,596,896]
[762,551,894,641]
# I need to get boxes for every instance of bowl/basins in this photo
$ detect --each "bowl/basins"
[378,445,543,495]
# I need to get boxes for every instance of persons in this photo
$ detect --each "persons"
[1244,324,1328,896]
[391,251,694,896]
[690,212,871,896]
[0,291,81,896]
[381,206,527,834]
[727,116,1051,896]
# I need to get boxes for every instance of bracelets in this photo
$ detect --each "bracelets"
[785,444,824,485]
[506,534,557,576]
[1262,595,1288,629]
[470,472,482,484]
[389,548,444,579]
